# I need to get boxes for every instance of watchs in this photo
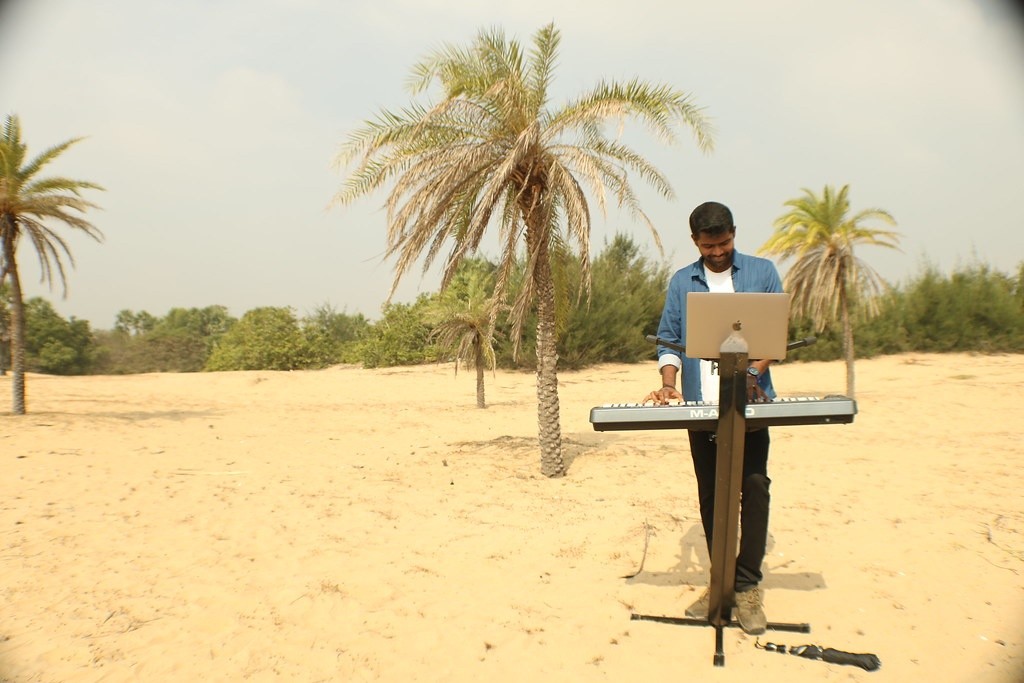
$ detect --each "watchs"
[747,366,761,384]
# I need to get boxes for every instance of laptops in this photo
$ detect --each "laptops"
[685,291,790,359]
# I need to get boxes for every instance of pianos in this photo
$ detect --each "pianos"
[589,394,859,432]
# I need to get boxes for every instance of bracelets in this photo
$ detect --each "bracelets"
[663,385,676,390]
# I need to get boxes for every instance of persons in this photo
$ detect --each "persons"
[643,202,783,636]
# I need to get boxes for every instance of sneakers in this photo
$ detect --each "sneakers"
[729,585,766,635]
[684,586,711,619]
